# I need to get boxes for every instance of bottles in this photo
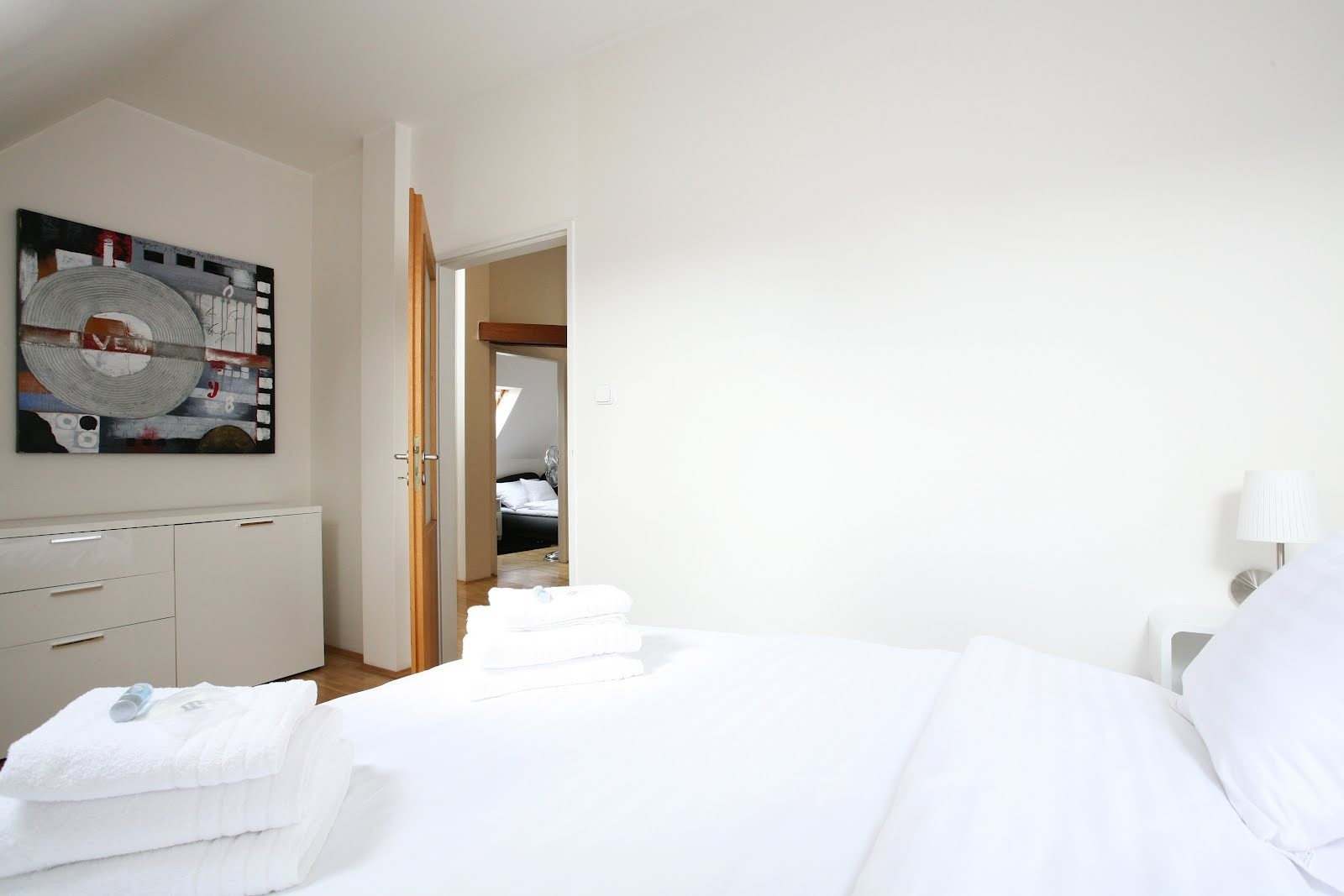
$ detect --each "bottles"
[109,683,154,722]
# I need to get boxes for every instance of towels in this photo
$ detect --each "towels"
[1,678,352,896]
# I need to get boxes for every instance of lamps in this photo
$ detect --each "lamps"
[1235,466,1325,577]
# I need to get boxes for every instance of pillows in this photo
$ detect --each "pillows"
[1181,542,1344,895]
[496,478,558,510]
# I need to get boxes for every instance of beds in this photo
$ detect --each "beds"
[0,623,1344,896]
[497,472,561,554]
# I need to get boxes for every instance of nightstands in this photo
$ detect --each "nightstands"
[1146,607,1238,684]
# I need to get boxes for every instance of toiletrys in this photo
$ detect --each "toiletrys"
[107,683,152,724]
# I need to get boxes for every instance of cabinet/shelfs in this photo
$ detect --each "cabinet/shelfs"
[174,508,324,688]
[0,526,175,764]
